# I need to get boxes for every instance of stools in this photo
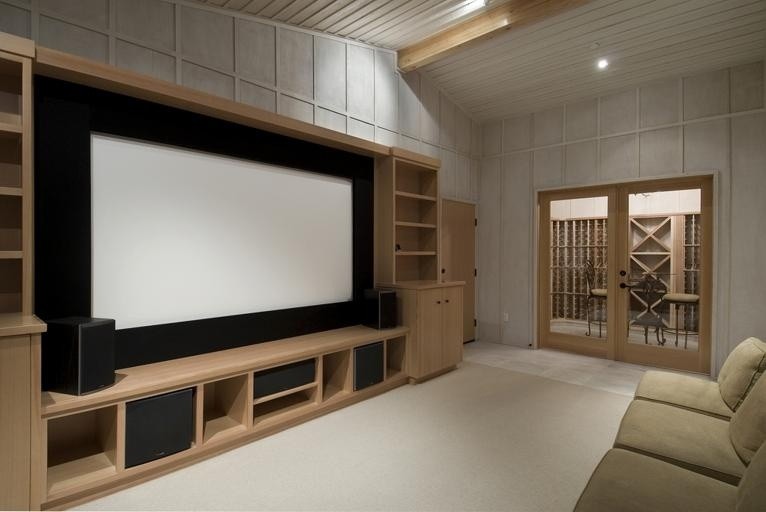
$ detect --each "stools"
[660,293,699,350]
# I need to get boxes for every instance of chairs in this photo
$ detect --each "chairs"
[583,259,608,338]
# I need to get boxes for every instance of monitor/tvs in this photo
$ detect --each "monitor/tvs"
[34,68,375,371]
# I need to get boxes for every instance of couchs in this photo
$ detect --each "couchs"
[574,335,766,512]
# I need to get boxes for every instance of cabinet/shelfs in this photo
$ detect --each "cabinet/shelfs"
[681,212,700,335]
[1,317,46,512]
[379,148,440,284]
[41,326,413,512]
[546,218,609,327]
[626,214,677,333]
[0,32,36,318]
[378,279,465,386]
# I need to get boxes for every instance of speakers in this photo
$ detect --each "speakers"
[254,358,315,398]
[41,316,115,396]
[125,389,193,468]
[353,342,384,391]
[364,288,396,329]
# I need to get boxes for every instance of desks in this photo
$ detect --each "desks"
[624,272,677,346]
